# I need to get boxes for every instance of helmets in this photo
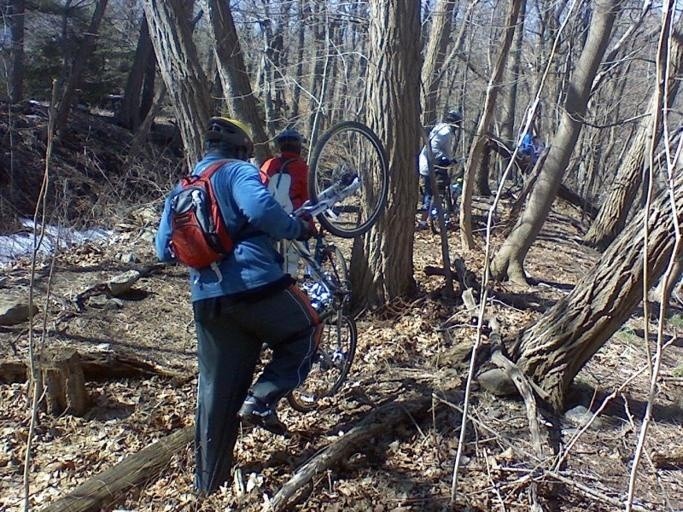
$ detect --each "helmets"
[446,109,463,120]
[204,115,254,156]
[276,129,303,148]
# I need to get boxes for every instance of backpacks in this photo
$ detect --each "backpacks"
[166,159,234,270]
[265,157,295,215]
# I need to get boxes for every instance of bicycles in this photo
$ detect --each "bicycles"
[278,121,390,412]
[429,156,465,234]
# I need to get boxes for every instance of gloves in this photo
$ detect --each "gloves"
[298,212,314,243]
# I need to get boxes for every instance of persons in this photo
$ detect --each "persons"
[529,136,545,176]
[257,130,322,287]
[153,116,324,498]
[516,124,532,156]
[413,109,463,233]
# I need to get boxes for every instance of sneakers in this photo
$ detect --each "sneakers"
[417,220,429,228]
[238,395,287,436]
